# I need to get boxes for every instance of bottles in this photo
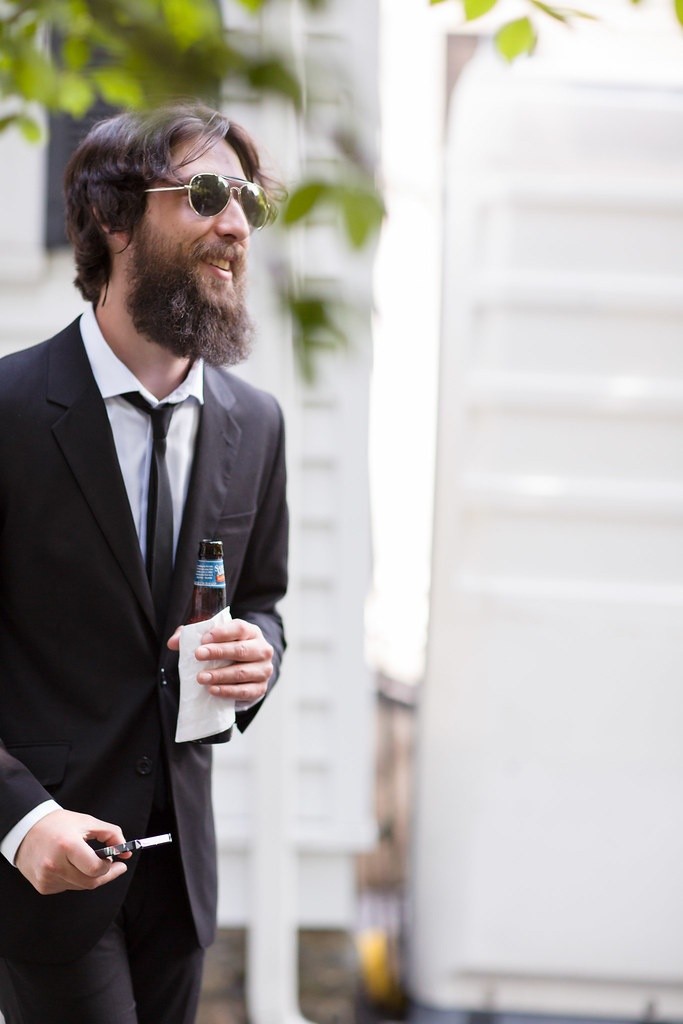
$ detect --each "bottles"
[174,537,233,745]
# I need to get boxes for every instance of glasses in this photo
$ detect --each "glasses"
[141,172,271,230]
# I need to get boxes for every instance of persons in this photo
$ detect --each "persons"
[0,105,289,1024]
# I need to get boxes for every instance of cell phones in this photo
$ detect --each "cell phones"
[96,834,174,859]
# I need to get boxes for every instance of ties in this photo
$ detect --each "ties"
[120,392,189,641]
